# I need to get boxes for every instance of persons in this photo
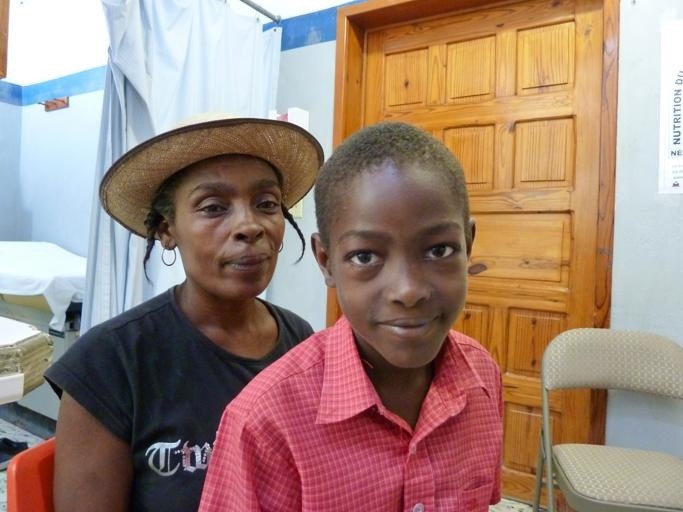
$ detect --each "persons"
[51,118,316,512]
[198,122,504,512]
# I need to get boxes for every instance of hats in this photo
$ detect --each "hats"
[101,111,325,241]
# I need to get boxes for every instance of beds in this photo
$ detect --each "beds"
[0,239,85,404]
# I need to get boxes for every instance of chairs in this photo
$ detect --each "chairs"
[535,327,683,512]
